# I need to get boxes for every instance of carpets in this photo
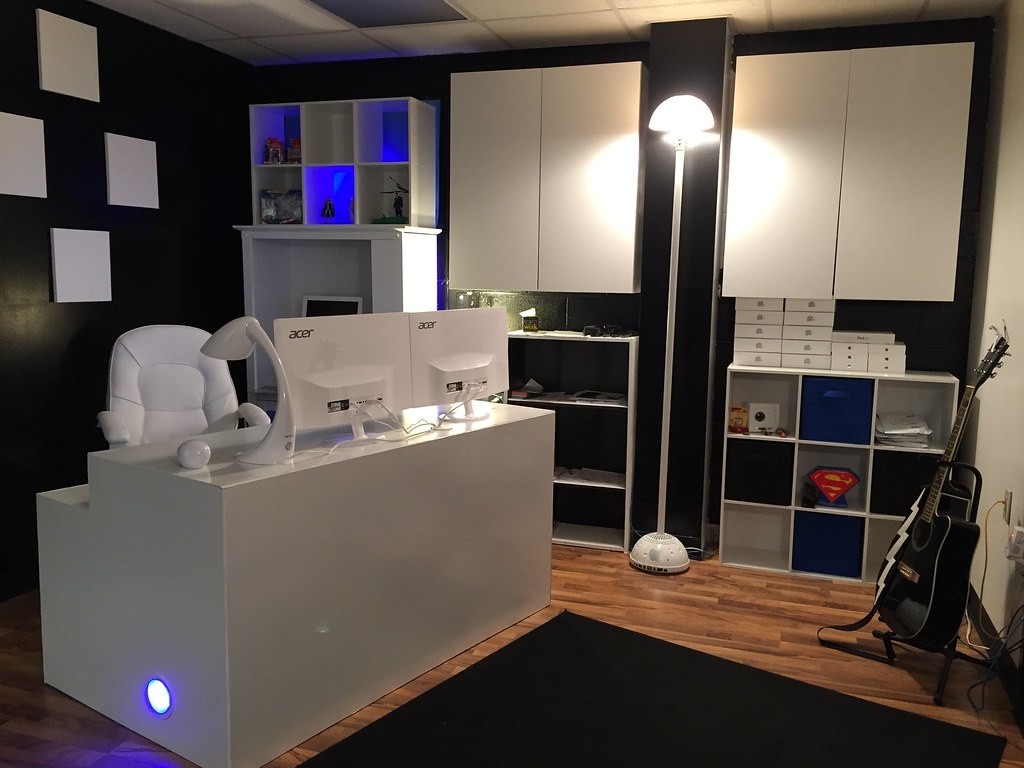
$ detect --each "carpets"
[295,612,1008,767]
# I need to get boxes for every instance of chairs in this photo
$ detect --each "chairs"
[96,324,271,450]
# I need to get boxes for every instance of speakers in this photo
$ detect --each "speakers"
[177,439,210,469]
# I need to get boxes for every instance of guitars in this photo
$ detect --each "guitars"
[874,335,1011,652]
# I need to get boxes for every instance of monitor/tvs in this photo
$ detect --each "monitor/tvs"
[273,311,414,450]
[302,296,362,318]
[409,305,509,422]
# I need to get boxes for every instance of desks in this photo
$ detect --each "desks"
[35,400,556,768]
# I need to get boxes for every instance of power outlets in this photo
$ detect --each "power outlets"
[1003,488,1013,525]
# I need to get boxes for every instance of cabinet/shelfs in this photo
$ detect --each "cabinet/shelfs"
[721,42,984,302]
[504,329,640,554]
[234,225,443,428]
[717,361,960,587]
[248,96,436,227]
[449,60,650,293]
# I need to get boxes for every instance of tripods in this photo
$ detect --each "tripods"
[872,629,1002,708]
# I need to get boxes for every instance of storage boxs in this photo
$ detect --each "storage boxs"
[733,297,906,374]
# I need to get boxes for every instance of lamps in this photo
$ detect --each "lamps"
[628,95,715,575]
